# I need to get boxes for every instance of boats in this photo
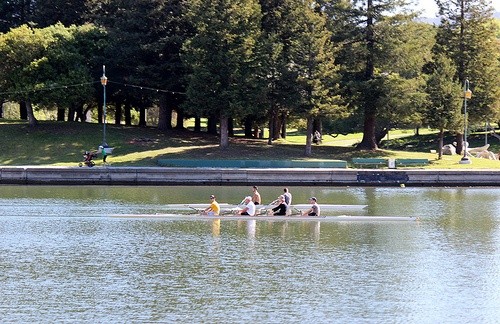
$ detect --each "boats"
[192,206,323,219]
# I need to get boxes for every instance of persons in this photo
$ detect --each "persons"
[269,195,288,216]
[302,196,320,216]
[96,145,109,166]
[238,196,255,216]
[252,185,262,205]
[246,221,256,245]
[203,194,220,216]
[212,218,221,252]
[283,188,293,205]
[313,130,321,145]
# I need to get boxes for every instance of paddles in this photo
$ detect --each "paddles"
[239,196,247,205]
[294,208,301,213]
[184,205,199,213]
[268,198,279,205]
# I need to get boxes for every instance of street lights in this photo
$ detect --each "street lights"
[459,77,472,161]
[100,65,107,145]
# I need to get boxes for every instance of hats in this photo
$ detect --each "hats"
[209,195,216,199]
[98,146,104,149]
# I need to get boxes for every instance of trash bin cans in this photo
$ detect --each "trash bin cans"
[388,158,395,168]
